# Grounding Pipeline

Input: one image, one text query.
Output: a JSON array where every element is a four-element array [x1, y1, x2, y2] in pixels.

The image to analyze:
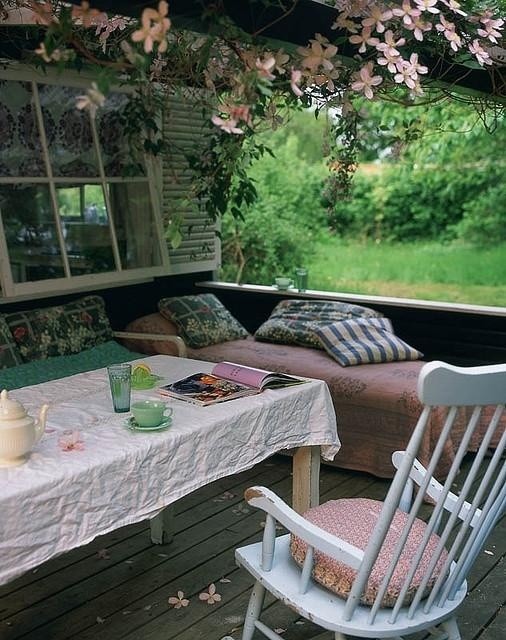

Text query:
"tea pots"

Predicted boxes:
[[1, 389, 50, 470]]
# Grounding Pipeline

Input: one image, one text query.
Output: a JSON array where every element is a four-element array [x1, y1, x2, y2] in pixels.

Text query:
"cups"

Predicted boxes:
[[130, 399, 173, 428], [107, 363, 132, 413]]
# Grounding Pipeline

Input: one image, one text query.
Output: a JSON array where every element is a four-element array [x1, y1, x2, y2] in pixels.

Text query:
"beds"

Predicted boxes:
[[121, 311, 506, 487]]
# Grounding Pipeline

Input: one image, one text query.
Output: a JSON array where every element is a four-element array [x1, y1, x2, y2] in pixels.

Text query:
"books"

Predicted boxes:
[[157, 361, 313, 408]]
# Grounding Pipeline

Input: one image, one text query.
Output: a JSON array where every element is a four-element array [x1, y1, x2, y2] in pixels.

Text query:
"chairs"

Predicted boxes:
[[0, 294, 186, 387], [220, 360, 506, 639]]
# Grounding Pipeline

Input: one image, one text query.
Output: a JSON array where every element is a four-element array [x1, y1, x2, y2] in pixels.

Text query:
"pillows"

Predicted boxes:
[[0, 295, 113, 368], [313, 316, 425, 368], [155, 292, 248, 349]]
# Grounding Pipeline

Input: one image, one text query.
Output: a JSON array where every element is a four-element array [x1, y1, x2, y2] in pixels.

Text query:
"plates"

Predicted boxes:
[[125, 416, 174, 432]]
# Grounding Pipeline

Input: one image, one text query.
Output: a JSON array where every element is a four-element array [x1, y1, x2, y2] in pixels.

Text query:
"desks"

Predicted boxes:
[[0, 355, 329, 546]]
